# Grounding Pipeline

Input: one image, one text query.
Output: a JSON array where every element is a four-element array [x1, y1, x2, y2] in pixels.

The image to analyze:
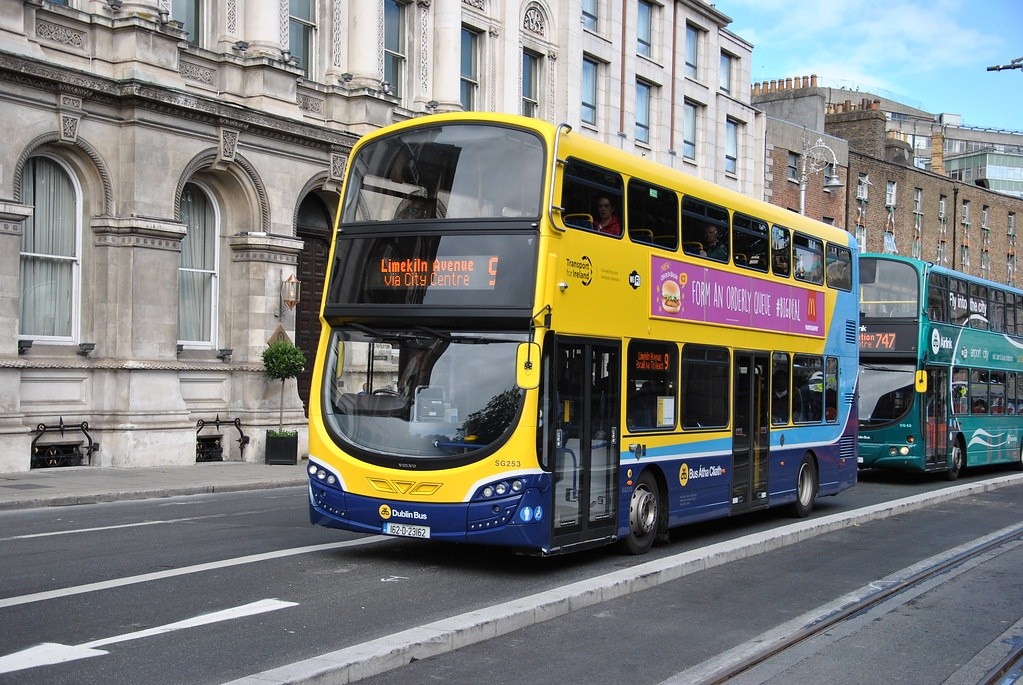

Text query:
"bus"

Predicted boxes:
[[305, 107, 862, 559], [808, 252, 1023, 481]]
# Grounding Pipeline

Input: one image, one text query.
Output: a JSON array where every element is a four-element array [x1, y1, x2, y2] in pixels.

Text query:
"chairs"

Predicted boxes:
[[566, 213, 768, 270]]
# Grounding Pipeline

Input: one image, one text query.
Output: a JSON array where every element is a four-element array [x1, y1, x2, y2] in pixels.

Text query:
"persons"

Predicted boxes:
[[401, 196, 434, 221], [592, 194, 621, 236], [602, 362, 675, 424], [754, 370, 802, 422], [699, 225, 729, 262]]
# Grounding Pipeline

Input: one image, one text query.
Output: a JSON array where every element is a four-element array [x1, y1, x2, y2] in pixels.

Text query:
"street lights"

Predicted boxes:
[[796, 136, 846, 218], [950, 177, 991, 271]]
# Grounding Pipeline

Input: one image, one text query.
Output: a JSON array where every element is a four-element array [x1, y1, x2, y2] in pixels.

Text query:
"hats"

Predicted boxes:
[[799, 370, 812, 383]]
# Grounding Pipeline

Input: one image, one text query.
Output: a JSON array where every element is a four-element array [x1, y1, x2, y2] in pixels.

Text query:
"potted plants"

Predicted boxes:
[[261, 341, 307, 465]]
